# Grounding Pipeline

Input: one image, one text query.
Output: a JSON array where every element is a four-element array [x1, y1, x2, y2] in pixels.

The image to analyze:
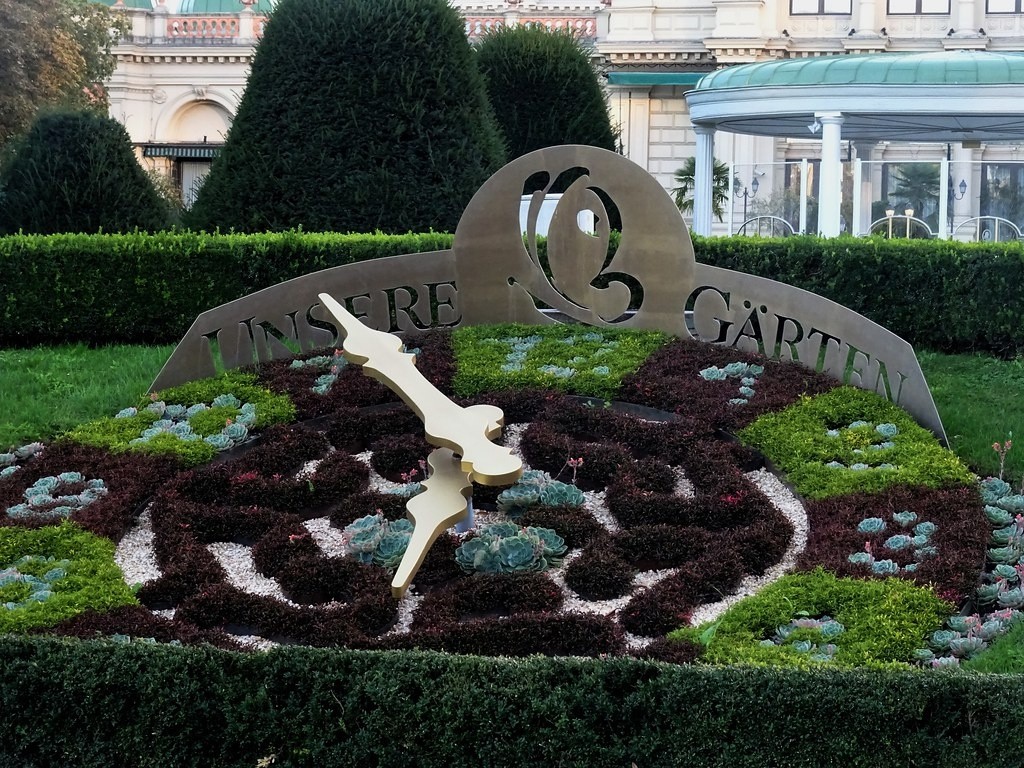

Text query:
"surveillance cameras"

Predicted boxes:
[[754, 169, 765, 177]]
